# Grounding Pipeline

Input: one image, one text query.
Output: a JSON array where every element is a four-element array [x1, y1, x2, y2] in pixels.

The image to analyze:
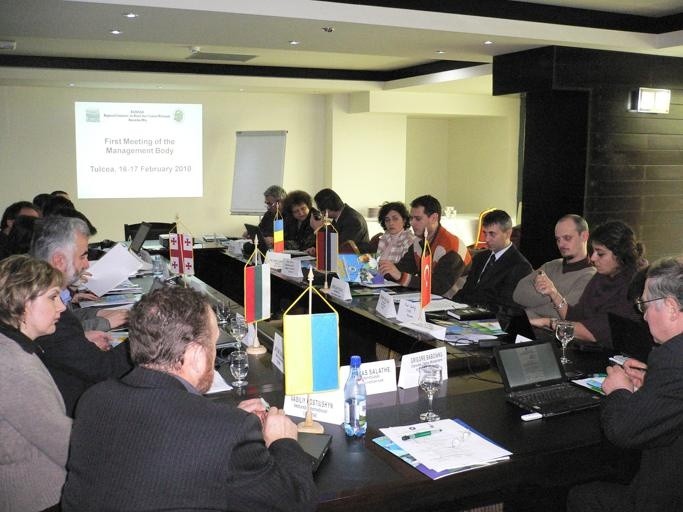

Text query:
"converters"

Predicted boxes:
[[478, 340, 502, 347]]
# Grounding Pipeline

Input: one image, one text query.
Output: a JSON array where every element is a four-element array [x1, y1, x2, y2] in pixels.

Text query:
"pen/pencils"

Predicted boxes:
[[586, 372, 607, 377], [628, 366, 648, 372], [403, 429, 442, 441], [259, 395, 271, 411], [535, 271, 544, 275]]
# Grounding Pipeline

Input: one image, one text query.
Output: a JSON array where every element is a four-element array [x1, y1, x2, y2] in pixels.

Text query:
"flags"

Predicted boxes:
[[169, 221, 195, 276], [244, 248, 271, 325], [420, 242, 431, 308], [283, 286, 340, 395], [313, 221, 339, 273]]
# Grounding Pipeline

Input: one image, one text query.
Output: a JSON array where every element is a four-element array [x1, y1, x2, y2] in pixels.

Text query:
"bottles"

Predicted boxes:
[[151, 251, 163, 277], [343, 356, 367, 438]]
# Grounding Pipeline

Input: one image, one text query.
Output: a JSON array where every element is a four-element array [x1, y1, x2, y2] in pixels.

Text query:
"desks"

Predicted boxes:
[[88, 236, 251, 285], [222, 242, 571, 355], [203, 348, 660, 512], [57, 254, 339, 401]]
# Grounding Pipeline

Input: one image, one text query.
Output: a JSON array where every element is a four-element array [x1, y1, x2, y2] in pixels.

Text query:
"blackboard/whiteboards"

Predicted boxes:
[[226, 128, 288, 216]]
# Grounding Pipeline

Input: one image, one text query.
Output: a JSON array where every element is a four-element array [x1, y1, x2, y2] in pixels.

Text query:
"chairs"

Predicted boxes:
[[124, 222, 177, 239]]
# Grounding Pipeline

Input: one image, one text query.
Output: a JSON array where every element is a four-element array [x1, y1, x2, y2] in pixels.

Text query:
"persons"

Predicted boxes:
[[565, 257, 683, 512], [452, 209, 535, 312], [61, 288, 319, 512], [530, 220, 656, 365], [513, 215, 598, 333], [1, 191, 133, 420], [244, 186, 369, 256], [379, 195, 472, 300], [0, 254, 73, 511], [372, 201, 418, 282]]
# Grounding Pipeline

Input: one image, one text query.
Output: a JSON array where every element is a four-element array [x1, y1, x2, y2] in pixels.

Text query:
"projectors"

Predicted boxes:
[[159, 234, 194, 248]]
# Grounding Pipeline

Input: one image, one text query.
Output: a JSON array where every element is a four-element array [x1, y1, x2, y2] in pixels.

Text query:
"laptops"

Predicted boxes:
[[244, 223, 268, 250], [129, 222, 153, 254], [492, 338, 606, 419], [486, 299, 556, 340]]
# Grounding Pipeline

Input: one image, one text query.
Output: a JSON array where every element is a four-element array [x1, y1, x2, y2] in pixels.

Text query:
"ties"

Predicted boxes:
[[480, 254, 495, 281]]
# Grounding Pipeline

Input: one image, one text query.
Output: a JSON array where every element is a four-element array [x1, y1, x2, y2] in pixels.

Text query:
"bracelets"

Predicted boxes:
[[554, 298, 567, 312], [550, 317, 560, 331]]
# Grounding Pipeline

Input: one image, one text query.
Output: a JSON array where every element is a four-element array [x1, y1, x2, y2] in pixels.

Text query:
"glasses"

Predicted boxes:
[[263, 201, 277, 209], [634, 296, 666, 312]]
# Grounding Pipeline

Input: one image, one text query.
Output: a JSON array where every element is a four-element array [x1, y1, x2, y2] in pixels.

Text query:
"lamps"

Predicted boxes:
[[628, 86, 671, 115]]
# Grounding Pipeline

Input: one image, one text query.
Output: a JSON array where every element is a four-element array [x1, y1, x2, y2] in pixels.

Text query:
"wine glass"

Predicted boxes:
[[230, 317, 249, 349], [217, 298, 232, 323], [419, 363, 443, 422], [229, 350, 249, 387], [555, 321, 576, 364]]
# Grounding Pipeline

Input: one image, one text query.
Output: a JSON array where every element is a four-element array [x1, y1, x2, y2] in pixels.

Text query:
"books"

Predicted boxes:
[[446, 306, 497, 320]]
